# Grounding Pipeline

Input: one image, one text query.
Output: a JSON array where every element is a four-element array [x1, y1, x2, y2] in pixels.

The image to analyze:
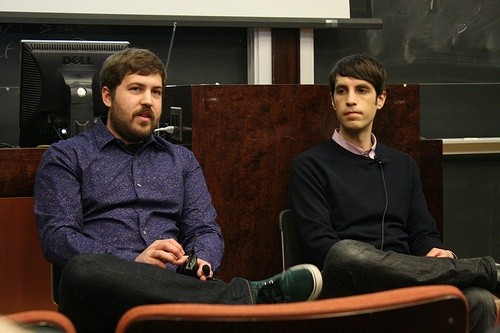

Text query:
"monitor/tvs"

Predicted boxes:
[[18, 40, 130, 147]]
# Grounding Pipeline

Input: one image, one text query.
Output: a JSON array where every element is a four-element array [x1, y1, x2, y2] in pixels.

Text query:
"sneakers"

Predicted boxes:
[[251, 264, 323, 305]]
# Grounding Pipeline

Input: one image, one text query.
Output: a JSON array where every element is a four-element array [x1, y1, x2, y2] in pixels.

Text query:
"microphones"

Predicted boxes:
[[203, 265, 210, 277], [378, 158, 389, 166], [165, 22, 177, 72]]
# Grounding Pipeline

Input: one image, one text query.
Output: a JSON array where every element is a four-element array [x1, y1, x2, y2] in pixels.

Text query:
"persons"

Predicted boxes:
[[289, 54, 500, 333], [34, 48, 324, 333]]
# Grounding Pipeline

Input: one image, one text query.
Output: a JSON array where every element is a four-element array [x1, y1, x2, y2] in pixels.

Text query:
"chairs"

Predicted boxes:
[[115, 285, 470, 333], [277, 209, 312, 274]]
